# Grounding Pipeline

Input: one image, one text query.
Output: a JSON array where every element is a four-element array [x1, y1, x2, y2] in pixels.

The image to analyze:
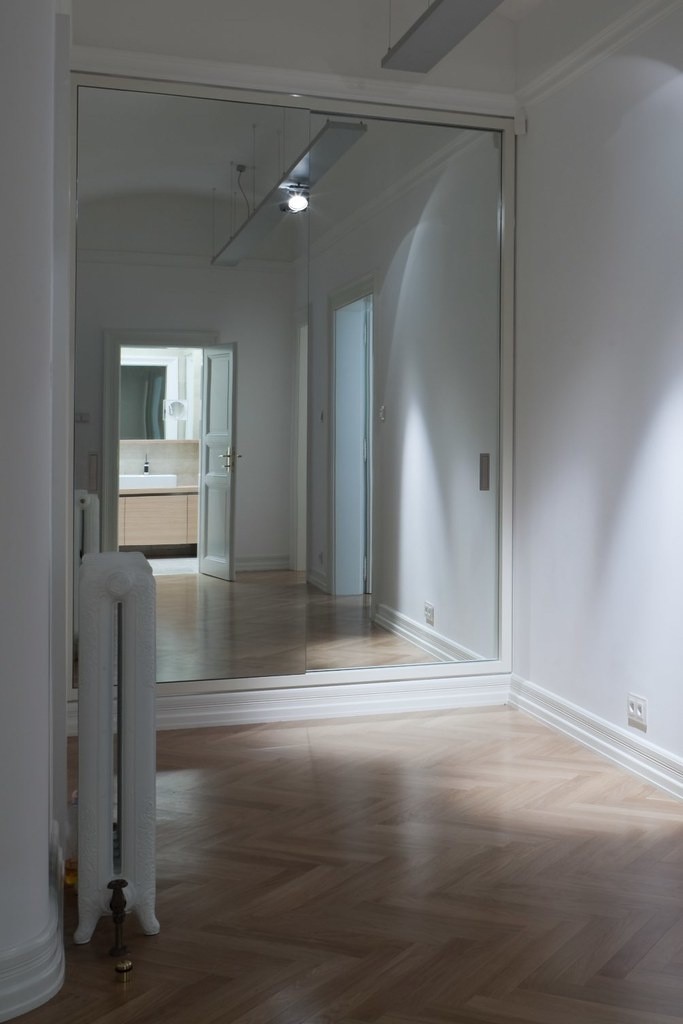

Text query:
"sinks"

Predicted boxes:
[[119, 475, 178, 490]]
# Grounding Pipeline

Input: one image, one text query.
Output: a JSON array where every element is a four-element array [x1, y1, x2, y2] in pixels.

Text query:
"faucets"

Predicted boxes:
[[143, 452, 150, 475]]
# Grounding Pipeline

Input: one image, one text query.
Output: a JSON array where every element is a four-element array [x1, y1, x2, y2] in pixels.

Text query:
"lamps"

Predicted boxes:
[[210, 106, 368, 268]]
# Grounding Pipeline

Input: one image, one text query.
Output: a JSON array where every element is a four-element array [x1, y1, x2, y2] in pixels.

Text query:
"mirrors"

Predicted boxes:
[[71, 69, 502, 687], [120, 366, 167, 440]]
